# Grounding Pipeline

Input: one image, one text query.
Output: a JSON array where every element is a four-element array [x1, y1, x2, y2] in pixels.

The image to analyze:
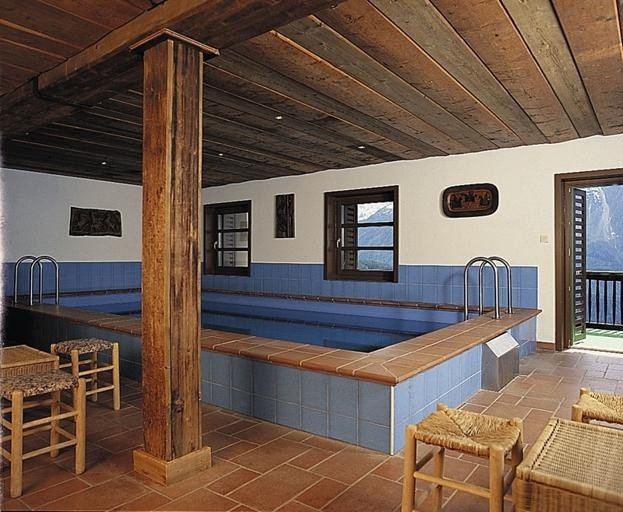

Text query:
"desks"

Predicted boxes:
[[1, 343, 60, 380], [512, 416, 623, 511]]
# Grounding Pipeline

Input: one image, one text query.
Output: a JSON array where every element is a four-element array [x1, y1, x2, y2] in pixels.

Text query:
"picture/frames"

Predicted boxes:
[[442, 182, 498, 217]]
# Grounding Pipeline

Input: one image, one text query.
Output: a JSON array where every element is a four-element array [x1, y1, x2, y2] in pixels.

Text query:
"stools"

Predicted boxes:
[[571, 385, 623, 424], [1, 369, 86, 499], [50, 338, 123, 411], [401, 404, 522, 512]]
